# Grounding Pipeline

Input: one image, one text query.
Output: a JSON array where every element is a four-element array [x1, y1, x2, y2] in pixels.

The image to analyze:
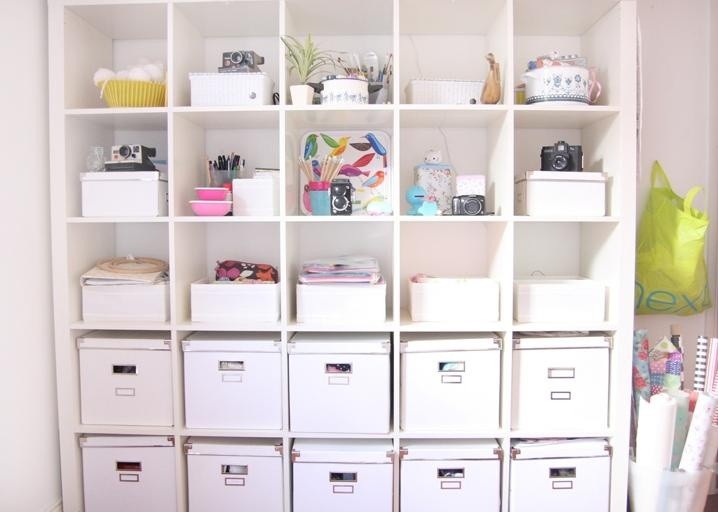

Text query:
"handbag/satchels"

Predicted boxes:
[[634, 156, 713, 316]]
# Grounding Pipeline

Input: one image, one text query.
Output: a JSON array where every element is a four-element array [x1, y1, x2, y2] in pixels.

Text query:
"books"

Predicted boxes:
[[79, 260, 162, 284]]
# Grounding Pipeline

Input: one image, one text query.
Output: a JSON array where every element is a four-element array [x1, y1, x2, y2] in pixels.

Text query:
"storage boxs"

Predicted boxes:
[[79, 171, 168, 217], [232, 178, 280, 216], [514, 170, 607, 216]]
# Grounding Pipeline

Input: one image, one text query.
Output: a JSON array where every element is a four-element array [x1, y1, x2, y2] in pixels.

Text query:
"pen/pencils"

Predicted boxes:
[[338, 54, 393, 80], [209, 151, 246, 171], [300, 156, 343, 182]]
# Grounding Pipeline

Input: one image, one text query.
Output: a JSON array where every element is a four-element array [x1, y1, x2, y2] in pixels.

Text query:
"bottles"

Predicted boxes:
[[224, 184, 232, 200]]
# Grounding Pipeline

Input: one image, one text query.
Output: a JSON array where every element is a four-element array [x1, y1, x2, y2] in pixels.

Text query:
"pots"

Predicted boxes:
[[520, 65, 594, 107], [303, 77, 384, 106]]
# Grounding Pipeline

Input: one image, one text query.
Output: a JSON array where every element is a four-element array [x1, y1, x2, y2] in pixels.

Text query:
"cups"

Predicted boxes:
[[309, 181, 329, 191], [311, 191, 332, 216]]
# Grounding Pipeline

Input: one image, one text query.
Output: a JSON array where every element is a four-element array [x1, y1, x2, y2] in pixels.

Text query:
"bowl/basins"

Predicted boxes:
[[195, 187, 227, 200], [188, 200, 233, 216]]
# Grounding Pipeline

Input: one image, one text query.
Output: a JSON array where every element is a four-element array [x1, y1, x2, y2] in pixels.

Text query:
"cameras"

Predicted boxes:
[[222, 51, 266, 66], [539, 140, 584, 172], [329, 178, 353, 215], [111, 144, 156, 164], [451, 194, 485, 216]]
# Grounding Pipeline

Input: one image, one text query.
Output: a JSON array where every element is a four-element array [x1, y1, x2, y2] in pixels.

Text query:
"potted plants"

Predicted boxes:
[[280, 33, 349, 105]]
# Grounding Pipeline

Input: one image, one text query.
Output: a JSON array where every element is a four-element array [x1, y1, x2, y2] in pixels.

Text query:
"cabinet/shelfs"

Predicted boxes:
[[48, 0, 638, 512]]
